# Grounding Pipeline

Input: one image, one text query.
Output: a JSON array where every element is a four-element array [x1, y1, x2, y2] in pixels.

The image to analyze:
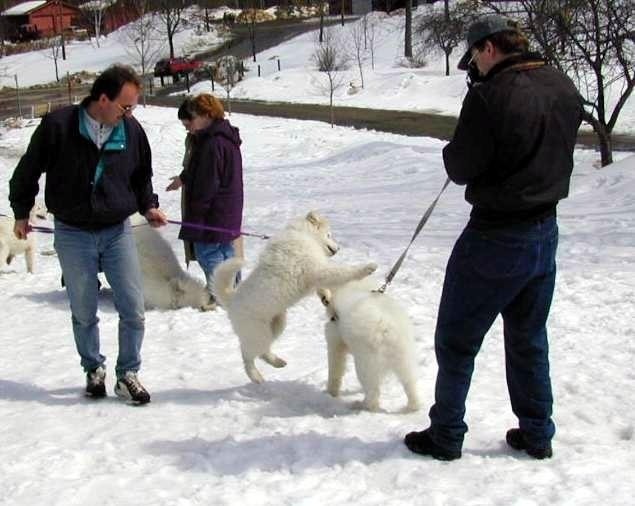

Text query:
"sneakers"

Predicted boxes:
[[405, 430, 461, 460], [506, 428, 553, 460], [115, 370, 151, 404], [84, 366, 108, 399]]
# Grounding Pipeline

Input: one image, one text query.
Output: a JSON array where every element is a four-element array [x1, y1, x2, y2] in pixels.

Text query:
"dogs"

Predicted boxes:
[[128, 211, 207, 312], [208, 210, 377, 385], [0, 200, 49, 275], [321, 269, 423, 413]]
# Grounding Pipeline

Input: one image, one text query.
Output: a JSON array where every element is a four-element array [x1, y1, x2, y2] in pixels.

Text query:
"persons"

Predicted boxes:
[[9, 61, 167, 407], [166, 93, 245, 314], [403, 15, 588, 465], [177, 94, 245, 287]]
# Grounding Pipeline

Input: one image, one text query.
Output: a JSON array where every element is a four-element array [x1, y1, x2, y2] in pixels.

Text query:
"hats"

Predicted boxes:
[[456, 14, 522, 72]]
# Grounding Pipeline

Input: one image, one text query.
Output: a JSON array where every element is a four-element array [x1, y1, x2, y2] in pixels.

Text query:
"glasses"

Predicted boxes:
[[110, 98, 137, 113], [466, 46, 481, 71]]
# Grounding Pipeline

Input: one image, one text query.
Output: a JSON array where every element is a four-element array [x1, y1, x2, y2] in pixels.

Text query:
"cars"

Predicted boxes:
[[154, 56, 203, 80]]
